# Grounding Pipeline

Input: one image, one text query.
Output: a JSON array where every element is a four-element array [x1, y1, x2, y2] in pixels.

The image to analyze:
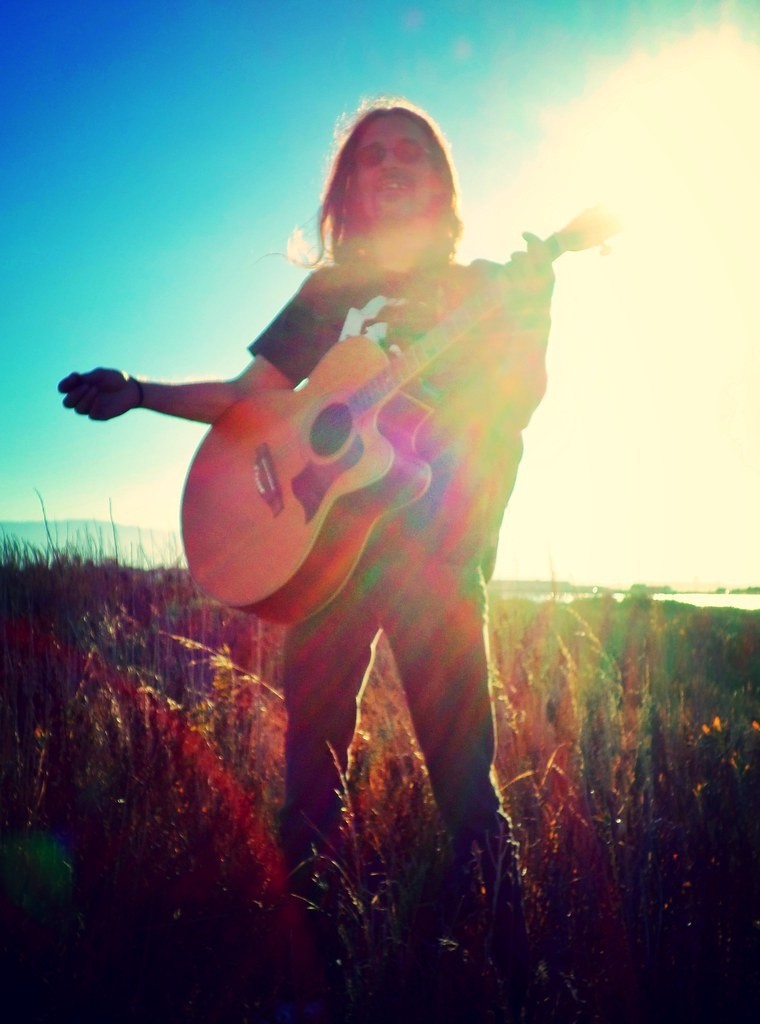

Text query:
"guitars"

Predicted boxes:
[[178, 200, 628, 623]]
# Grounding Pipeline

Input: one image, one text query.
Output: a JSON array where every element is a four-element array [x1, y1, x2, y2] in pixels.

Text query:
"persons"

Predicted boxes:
[[58, 102, 555, 1024]]
